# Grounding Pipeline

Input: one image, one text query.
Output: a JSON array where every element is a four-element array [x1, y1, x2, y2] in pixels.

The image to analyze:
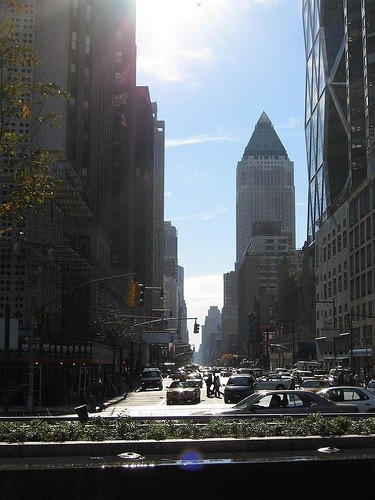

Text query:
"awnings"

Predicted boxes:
[[348, 348, 373, 357]]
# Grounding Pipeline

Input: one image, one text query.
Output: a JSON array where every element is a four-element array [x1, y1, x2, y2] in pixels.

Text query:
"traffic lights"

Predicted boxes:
[[127, 281, 145, 307], [194, 323, 199, 333]]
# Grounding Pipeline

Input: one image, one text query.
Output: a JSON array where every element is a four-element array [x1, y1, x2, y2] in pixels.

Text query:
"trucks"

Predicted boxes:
[[296, 360, 323, 371]]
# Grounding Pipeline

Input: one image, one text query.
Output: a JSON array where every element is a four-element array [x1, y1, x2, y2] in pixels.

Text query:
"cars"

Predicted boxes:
[[165, 368, 204, 404], [139, 368, 164, 390], [190, 389, 359, 420], [214, 366, 375, 414]]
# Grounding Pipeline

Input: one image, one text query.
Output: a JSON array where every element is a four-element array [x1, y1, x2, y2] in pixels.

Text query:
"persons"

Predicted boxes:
[[368, 377, 375, 388], [90, 375, 106, 413], [288, 378, 295, 401], [262, 395, 281, 408], [338, 372, 360, 386], [204, 372, 220, 398]]
